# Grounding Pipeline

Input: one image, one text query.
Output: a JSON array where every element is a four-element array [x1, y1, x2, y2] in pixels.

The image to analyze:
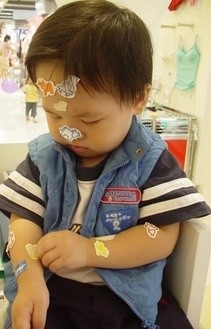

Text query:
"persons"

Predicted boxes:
[[21, 74, 40, 122], [12, 50, 27, 88], [0, 35, 13, 80], [0, 0, 210, 329]]
